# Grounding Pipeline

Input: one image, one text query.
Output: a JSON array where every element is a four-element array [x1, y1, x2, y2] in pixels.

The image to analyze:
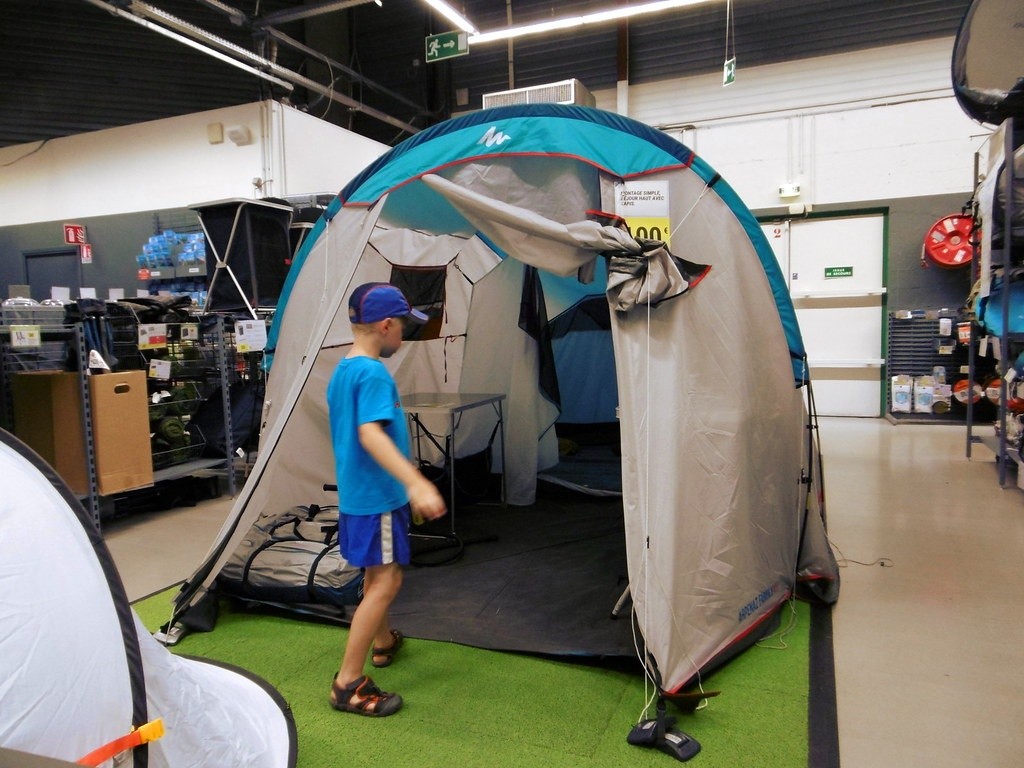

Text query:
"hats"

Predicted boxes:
[[349, 281, 427, 324]]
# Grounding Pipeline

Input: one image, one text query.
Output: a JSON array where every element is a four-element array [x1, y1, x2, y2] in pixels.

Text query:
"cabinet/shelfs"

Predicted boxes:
[[0, 294, 277, 529], [136, 212, 208, 294], [965, 119, 1024, 490], [888, 313, 997, 425]]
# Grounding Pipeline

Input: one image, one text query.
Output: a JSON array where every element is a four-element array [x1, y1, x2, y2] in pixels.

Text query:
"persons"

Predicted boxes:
[[327, 283, 450, 719]]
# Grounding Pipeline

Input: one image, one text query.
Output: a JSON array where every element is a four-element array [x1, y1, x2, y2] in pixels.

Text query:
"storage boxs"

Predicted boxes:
[[187, 198, 293, 312], [289, 204, 328, 258]]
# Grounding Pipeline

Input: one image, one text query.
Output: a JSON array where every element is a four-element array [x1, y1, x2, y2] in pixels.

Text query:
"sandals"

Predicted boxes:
[[329, 668, 403, 718], [371, 625, 404, 668]]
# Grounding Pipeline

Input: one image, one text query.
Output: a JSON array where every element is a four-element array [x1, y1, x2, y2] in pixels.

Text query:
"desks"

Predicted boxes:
[[398, 393, 509, 543]]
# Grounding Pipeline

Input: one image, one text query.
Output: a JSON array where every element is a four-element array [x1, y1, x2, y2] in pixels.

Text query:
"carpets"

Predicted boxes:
[[128, 580, 837, 768]]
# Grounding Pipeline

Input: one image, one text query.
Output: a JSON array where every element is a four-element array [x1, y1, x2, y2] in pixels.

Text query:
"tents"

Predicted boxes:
[[1, 427, 298, 768], [153, 104, 841, 763]]
